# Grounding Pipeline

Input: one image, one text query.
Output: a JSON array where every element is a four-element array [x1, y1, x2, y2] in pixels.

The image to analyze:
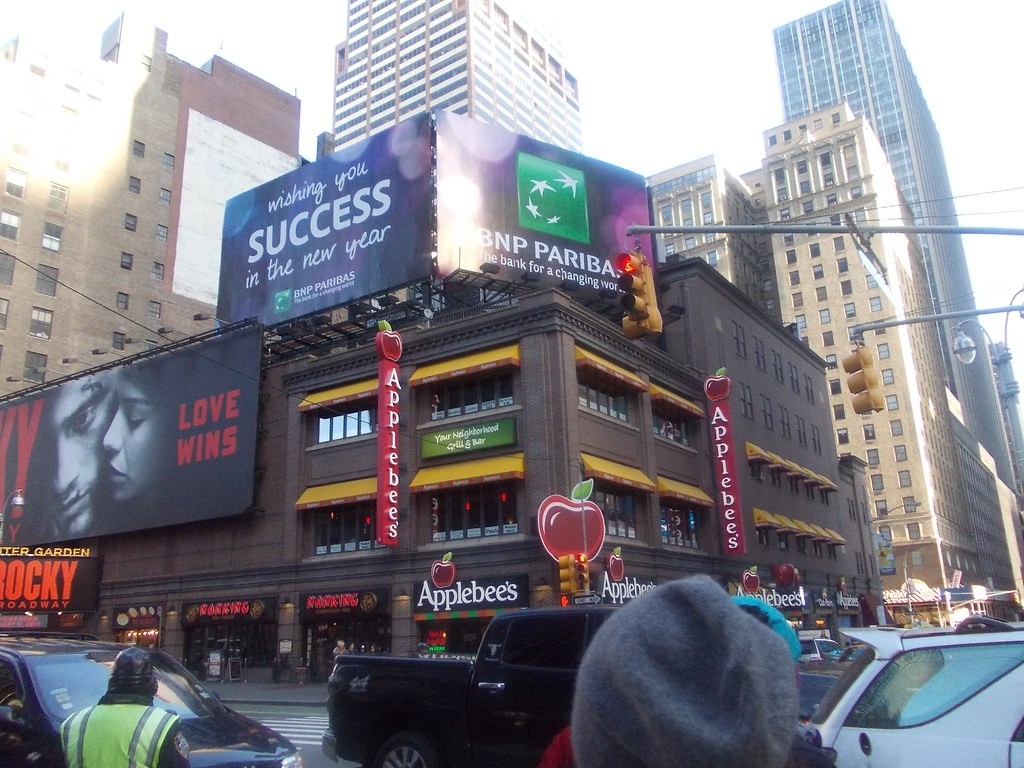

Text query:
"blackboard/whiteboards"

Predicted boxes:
[[226, 657, 242, 681]]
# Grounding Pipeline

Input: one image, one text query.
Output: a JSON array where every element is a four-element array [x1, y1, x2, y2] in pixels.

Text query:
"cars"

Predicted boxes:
[[796, 614, 1024, 767]]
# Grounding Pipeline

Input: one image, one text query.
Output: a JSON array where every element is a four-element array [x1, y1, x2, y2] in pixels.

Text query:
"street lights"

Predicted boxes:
[[949, 285, 1024, 513], [860, 484, 922, 627], [904, 535, 933, 623]]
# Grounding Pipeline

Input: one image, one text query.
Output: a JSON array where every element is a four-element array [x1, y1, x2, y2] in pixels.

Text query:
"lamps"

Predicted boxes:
[[157, 326, 192, 337], [5, 376, 40, 384], [91, 349, 126, 358], [62, 357, 96, 367], [558, 279, 579, 294], [125, 337, 161, 347], [466, 262, 500, 286], [276, 324, 297, 338], [311, 314, 332, 329], [349, 301, 371, 317], [377, 295, 408, 310], [584, 288, 618, 308], [33, 367, 68, 376], [192, 312, 236, 327], [662, 305, 685, 326], [500, 270, 541, 300]]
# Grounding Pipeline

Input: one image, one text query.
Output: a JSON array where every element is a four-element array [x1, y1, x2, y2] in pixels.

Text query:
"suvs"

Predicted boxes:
[[0, 626, 306, 768]]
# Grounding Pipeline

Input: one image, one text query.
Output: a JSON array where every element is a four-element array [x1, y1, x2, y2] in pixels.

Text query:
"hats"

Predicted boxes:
[[571, 572, 801, 768], [730, 593, 800, 663]]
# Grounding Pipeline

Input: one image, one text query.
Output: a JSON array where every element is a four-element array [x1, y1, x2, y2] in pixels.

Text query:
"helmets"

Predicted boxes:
[[108, 648, 157, 695]]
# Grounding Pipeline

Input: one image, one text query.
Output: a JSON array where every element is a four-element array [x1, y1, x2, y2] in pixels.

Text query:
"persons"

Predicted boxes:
[[820, 629, 828, 639], [370, 643, 376, 652], [539, 573, 839, 768], [332, 639, 349, 656], [42, 356, 225, 539], [411, 643, 428, 658], [59, 646, 191, 768], [347, 641, 359, 653]]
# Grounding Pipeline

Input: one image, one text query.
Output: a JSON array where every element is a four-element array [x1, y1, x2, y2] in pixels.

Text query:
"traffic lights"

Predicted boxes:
[[1018, 512, 1024, 542], [574, 554, 590, 589], [558, 554, 577, 595], [620, 264, 664, 341], [614, 250, 650, 320], [842, 346, 886, 415], [559, 594, 570, 607]]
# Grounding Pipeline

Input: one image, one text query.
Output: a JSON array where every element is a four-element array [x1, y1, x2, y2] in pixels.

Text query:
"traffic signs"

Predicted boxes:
[[573, 594, 602, 606]]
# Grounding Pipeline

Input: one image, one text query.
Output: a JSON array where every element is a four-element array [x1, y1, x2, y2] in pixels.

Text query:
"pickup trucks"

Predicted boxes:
[[322, 604, 854, 768]]
